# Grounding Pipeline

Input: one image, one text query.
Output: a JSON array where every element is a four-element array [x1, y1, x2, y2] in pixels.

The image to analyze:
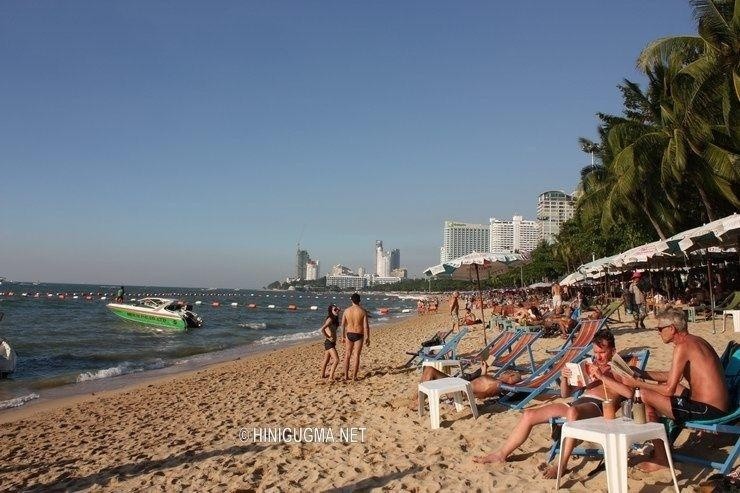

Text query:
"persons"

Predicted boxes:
[[448, 290, 462, 334], [414, 293, 440, 313], [320, 303, 341, 382], [621, 306, 730, 473], [341, 292, 371, 382], [116, 285, 125, 303], [471, 328, 635, 482], [407, 359, 523, 407], [459, 267, 740, 339]]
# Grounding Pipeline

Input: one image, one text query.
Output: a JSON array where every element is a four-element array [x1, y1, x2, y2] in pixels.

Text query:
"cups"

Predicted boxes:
[[602, 400, 615, 419]]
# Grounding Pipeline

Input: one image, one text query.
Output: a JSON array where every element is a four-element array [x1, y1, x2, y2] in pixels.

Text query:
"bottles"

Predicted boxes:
[[631, 386, 646, 424]]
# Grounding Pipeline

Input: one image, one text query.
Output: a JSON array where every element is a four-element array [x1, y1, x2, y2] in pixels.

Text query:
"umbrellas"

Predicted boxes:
[[558, 211, 740, 334], [422, 244, 533, 345]]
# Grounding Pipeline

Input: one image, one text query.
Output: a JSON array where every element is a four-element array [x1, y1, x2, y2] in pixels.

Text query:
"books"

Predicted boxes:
[[563, 356, 601, 390], [607, 351, 641, 380]]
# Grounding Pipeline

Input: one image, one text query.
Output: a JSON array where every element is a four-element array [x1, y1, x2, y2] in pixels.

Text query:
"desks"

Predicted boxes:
[[418, 377, 479, 428], [556, 416, 682, 493], [653, 304, 706, 323], [722, 310, 740, 333]]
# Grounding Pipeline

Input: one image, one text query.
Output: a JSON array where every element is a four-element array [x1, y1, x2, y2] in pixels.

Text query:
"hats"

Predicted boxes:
[[631, 272, 642, 279]]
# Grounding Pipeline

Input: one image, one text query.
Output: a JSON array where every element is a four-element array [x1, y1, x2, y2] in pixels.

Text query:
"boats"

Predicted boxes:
[[106, 298, 202, 333]]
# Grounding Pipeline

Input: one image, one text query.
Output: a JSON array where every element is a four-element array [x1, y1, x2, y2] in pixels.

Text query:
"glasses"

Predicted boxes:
[[334, 308, 339, 311], [657, 325, 671, 332]]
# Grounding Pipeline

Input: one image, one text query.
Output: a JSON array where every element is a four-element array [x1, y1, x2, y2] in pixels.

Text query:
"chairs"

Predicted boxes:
[[404, 317, 609, 410], [706, 292, 740, 321], [553, 301, 623, 336], [547, 350, 650, 476], [548, 304, 607, 335], [628, 340, 740, 476]]
